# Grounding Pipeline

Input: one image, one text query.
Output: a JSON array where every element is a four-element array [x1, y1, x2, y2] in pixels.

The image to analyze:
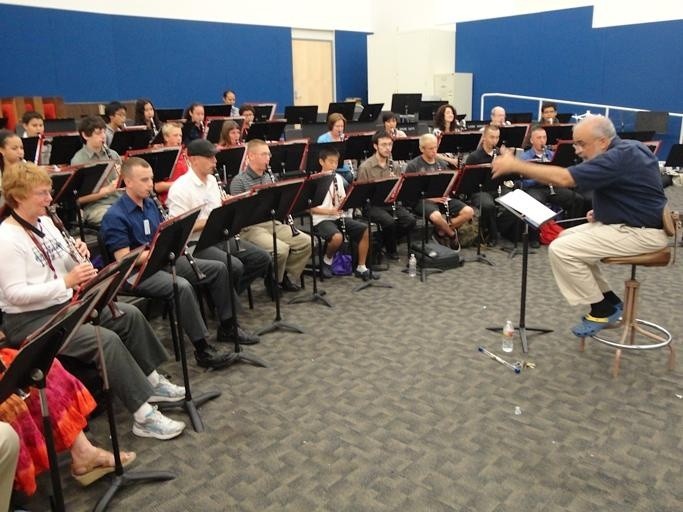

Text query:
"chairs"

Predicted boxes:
[[578, 203, 679, 378]]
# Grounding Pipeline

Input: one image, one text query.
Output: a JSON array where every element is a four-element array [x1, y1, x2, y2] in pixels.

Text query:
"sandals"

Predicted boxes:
[[571, 301, 623, 339]]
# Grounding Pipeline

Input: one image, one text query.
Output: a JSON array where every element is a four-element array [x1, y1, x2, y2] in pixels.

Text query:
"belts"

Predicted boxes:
[[627, 223, 656, 228]]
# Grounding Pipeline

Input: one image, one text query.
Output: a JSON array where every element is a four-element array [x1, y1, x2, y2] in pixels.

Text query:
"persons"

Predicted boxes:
[[466, 126, 516, 247], [519, 126, 584, 249], [430, 106, 466, 169], [19, 112, 48, 146], [491, 114, 669, 338], [97, 157, 261, 371], [102, 102, 128, 147], [524, 102, 565, 154], [0, 421, 20, 512], [0, 159, 189, 440], [230, 140, 313, 299], [165, 139, 274, 321], [0, 351, 135, 490], [182, 104, 206, 147], [382, 113, 408, 176], [0, 132, 25, 211], [222, 90, 241, 119], [69, 116, 127, 226], [136, 100, 163, 147], [478, 106, 512, 137], [215, 120, 249, 184], [317, 113, 354, 191], [237, 103, 256, 144], [357, 131, 413, 272], [406, 134, 474, 254], [153, 122, 189, 208], [305, 147, 371, 282]]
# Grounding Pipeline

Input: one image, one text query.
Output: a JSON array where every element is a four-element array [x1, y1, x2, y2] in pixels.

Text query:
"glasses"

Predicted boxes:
[[571, 137, 603, 149], [117, 113, 126, 116], [253, 152, 272, 158]]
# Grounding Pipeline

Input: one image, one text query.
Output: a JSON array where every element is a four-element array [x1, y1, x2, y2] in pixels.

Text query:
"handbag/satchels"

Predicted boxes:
[[538, 219, 565, 245]]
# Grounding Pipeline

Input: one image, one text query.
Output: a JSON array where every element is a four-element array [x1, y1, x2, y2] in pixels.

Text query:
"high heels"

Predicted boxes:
[[70, 448, 136, 487]]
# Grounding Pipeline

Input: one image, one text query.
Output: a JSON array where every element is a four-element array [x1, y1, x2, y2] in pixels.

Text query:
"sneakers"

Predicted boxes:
[[530, 241, 539, 248], [134, 380, 188, 440]]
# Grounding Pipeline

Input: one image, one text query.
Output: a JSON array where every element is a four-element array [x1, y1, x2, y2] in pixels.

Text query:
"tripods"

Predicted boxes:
[[92, 319, 176, 512], [147, 259, 221, 433], [351, 203, 394, 293], [401, 195, 443, 283], [288, 203, 336, 307], [486, 223, 554, 354], [252, 214, 306, 336], [465, 187, 496, 266], [203, 234, 271, 373], [500, 176, 537, 259]]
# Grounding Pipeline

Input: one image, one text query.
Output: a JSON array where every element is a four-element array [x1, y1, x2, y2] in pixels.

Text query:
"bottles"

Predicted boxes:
[[409, 254, 417, 277], [503, 319, 514, 352]]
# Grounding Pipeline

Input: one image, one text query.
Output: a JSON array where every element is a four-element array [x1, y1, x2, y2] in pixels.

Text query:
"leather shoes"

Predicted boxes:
[[194, 324, 261, 366], [267, 232, 461, 300]]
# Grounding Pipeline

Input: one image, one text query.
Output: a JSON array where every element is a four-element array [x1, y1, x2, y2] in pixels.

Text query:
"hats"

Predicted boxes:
[[189, 139, 218, 157]]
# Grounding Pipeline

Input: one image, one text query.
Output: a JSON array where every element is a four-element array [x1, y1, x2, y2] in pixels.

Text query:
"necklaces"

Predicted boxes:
[[19, 223, 57, 280]]
